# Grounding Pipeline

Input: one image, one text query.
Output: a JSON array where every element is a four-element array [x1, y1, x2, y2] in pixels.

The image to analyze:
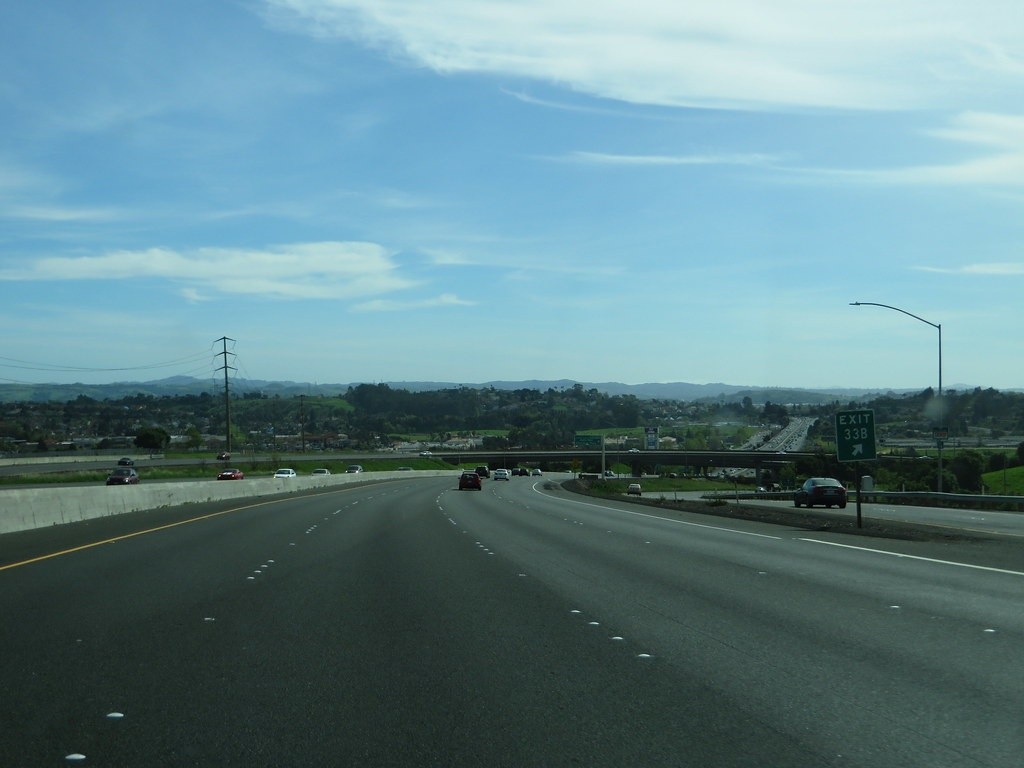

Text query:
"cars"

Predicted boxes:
[[272, 469, 296, 478], [532, 469, 542, 476], [312, 469, 330, 475], [119, 457, 134, 465], [756, 487, 766, 492], [458, 473, 482, 490], [105, 468, 139, 486], [513, 467, 531, 476], [217, 452, 231, 460], [398, 467, 414, 471], [494, 469, 511, 480], [217, 469, 243, 480], [345, 464, 362, 473], [629, 447, 640, 453], [794, 478, 846, 508], [420, 450, 432, 455]]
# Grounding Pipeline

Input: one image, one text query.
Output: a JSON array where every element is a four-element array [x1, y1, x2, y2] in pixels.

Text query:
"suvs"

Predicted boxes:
[[475, 466, 490, 478], [766, 483, 781, 492]]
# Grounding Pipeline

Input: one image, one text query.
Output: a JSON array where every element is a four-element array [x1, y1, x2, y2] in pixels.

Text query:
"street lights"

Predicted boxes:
[[851, 301, 942, 502]]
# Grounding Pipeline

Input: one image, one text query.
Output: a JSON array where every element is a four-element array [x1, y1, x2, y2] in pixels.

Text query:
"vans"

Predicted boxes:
[[627, 483, 641, 494]]
